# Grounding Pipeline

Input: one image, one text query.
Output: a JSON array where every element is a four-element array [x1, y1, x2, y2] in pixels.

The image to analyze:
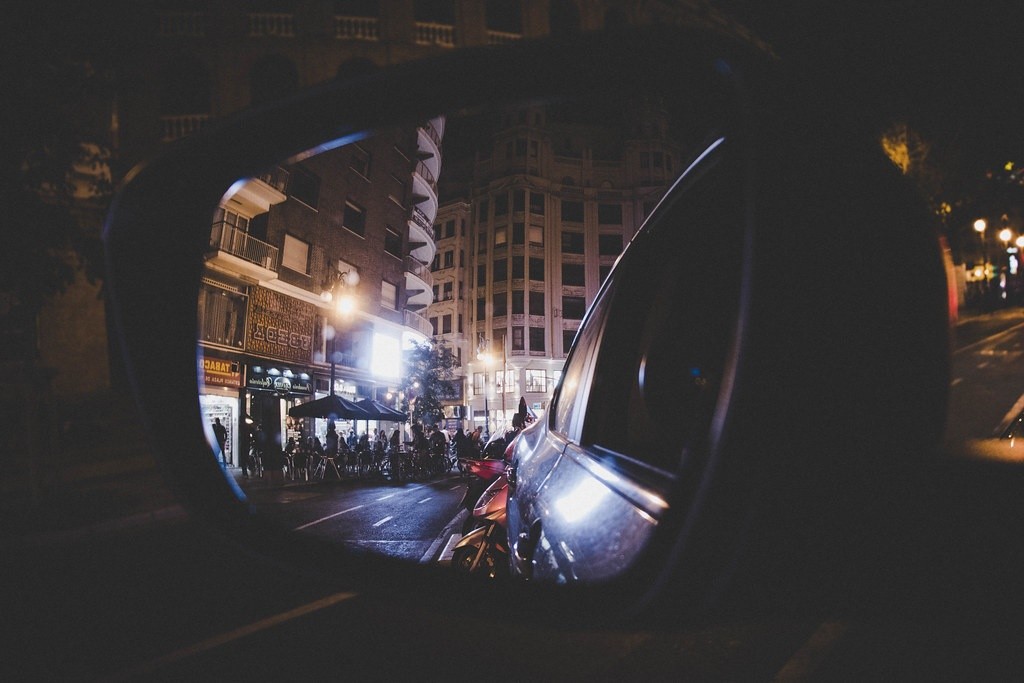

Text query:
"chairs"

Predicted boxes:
[[280, 450, 389, 482]]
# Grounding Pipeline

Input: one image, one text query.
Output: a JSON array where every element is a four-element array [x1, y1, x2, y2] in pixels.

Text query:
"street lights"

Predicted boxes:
[[319, 257, 350, 455], [475, 334, 493, 438]]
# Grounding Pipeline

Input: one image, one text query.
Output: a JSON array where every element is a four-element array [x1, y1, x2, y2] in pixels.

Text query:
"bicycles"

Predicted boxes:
[[346, 446, 465, 480]]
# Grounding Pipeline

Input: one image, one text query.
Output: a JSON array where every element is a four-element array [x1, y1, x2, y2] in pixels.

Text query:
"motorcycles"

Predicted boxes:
[[448, 468, 512, 582]]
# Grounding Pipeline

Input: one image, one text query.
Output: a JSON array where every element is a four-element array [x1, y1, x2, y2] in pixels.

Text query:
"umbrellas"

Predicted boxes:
[[352, 397, 409, 435], [288, 394, 371, 421]]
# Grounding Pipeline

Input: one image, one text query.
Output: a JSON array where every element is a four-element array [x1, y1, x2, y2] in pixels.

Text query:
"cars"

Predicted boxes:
[[463, 428, 516, 508]]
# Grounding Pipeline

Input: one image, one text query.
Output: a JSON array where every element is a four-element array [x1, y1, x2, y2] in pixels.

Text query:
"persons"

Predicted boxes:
[[238, 412, 485, 488], [211, 417, 227, 470]]
